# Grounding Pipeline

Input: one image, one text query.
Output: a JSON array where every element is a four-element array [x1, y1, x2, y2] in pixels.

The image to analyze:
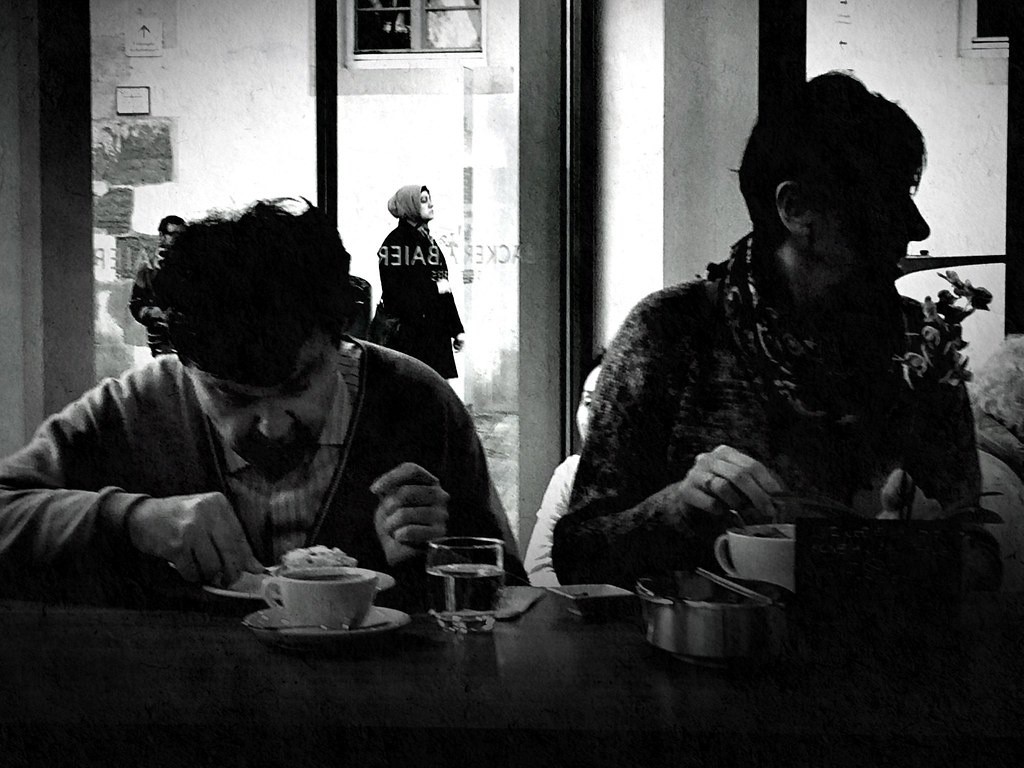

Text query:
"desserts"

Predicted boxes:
[[281, 546, 358, 572]]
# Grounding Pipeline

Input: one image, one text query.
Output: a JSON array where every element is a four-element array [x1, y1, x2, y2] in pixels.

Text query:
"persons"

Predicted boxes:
[[0, 195, 530, 621], [550, 71, 988, 588], [128, 215, 185, 359], [367, 184, 464, 380], [523, 344, 606, 589]]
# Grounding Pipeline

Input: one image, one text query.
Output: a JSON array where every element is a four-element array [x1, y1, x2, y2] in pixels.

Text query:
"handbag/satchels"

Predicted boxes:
[[368, 302, 405, 346]]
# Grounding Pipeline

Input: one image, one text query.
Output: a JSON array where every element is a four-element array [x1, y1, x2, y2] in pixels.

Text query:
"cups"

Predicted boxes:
[[425, 537, 507, 632], [714, 524, 797, 594], [261, 566, 380, 630]]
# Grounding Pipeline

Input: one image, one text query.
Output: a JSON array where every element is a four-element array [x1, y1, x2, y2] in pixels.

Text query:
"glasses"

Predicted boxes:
[[166, 230, 178, 236], [421, 195, 428, 202]]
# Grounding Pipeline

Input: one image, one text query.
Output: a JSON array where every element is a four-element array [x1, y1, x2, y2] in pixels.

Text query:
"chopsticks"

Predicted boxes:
[[694, 565, 774, 607]]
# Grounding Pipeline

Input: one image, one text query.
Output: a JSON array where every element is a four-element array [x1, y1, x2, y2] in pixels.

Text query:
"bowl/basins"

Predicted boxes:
[[635, 571, 792, 664]]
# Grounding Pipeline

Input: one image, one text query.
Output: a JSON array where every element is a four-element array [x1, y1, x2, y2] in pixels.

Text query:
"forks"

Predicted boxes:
[[242, 618, 392, 631]]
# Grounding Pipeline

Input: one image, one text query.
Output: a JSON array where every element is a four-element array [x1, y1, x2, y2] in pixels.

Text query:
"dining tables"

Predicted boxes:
[[0, 583, 1023, 738]]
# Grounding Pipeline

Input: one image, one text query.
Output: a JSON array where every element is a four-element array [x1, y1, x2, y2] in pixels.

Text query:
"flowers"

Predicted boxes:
[[770, 267, 1007, 592]]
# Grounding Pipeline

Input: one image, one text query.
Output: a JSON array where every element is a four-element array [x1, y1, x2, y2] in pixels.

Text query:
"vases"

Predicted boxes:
[[794, 514, 964, 668]]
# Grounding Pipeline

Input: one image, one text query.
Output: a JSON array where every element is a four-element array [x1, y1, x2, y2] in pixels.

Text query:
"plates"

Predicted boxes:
[[542, 583, 634, 622], [199, 565, 398, 607], [243, 605, 411, 649]]
